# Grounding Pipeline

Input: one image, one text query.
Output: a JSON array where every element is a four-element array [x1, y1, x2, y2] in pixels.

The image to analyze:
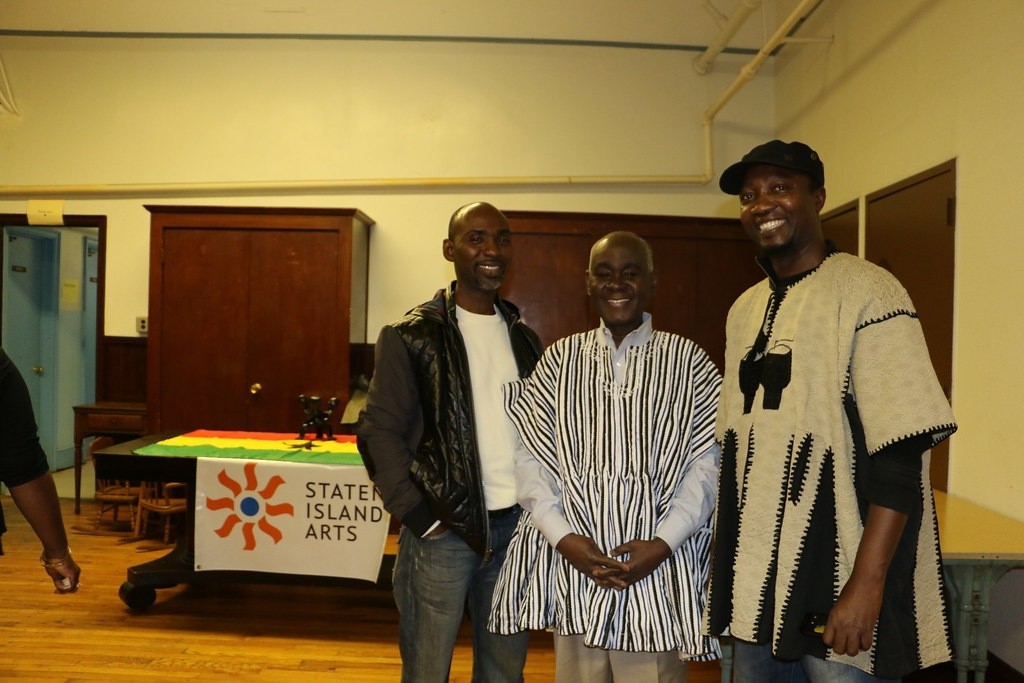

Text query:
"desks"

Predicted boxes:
[[927, 483, 1023, 682], [92, 426, 409, 609]]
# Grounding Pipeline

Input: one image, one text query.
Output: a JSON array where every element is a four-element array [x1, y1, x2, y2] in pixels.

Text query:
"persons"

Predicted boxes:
[[0, 346, 80, 594], [701, 140, 960, 683], [486, 232, 724, 683], [356, 201, 545, 683]]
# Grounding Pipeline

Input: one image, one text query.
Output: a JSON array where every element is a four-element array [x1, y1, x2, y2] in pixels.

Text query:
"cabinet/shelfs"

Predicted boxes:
[[74, 400, 146, 518], [488, 206, 776, 386], [143, 202, 377, 497]]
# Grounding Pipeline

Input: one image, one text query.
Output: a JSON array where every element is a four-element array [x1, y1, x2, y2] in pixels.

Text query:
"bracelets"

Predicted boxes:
[[39, 547, 72, 568]]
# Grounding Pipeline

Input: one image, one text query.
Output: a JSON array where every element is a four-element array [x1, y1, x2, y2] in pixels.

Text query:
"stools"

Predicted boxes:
[[89, 438, 140, 529], [133, 468, 188, 544]]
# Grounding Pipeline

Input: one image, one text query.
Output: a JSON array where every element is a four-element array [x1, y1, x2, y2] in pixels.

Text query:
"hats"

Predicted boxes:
[[719, 140, 825, 195]]
